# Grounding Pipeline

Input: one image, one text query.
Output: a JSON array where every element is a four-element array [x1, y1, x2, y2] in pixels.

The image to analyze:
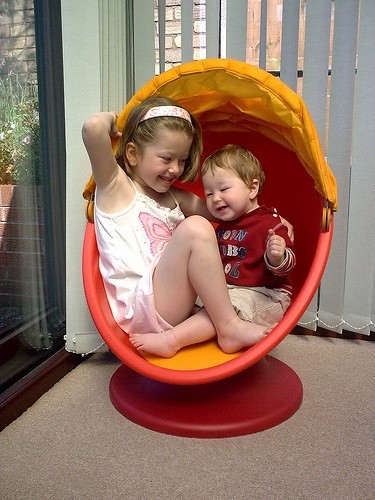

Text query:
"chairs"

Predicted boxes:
[[81, 58, 338, 438]]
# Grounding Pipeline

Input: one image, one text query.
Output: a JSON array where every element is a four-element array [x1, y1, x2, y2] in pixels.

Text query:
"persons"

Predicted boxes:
[[82, 94, 280, 354], [127, 144, 297, 360]]
